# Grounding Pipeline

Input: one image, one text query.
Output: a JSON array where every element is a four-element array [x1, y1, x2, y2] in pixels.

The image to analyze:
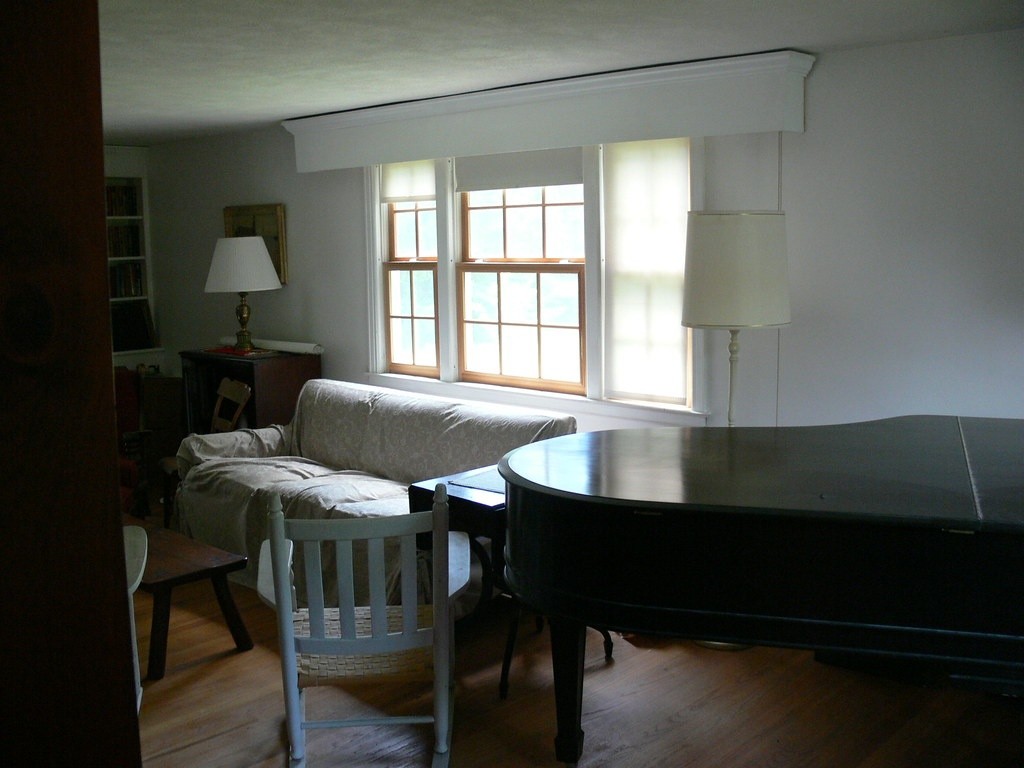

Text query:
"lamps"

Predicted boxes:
[[204, 236, 283, 350], [682, 210, 788, 426]]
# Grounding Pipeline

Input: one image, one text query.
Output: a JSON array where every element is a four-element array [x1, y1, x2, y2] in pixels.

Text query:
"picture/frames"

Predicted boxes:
[[222, 203, 288, 285]]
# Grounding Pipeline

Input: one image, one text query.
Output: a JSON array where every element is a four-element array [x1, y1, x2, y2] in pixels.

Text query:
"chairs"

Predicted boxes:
[[158, 377, 250, 527], [266, 484, 457, 767]]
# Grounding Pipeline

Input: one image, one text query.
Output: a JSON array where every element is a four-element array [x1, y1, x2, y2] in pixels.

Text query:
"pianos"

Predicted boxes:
[[497, 410, 1024, 763]]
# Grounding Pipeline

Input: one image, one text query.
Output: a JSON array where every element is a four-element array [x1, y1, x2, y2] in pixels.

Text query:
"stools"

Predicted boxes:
[[120, 509, 254, 680]]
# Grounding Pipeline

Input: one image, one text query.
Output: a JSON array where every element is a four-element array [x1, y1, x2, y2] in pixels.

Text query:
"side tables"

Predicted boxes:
[[410, 462, 614, 694]]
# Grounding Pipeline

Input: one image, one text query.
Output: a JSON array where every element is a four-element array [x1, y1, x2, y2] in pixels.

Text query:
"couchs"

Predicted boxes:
[[172, 378, 576, 626]]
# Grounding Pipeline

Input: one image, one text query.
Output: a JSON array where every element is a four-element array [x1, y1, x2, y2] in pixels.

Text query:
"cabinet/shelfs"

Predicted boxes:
[[179, 345, 321, 434], [104, 174, 163, 355]]
